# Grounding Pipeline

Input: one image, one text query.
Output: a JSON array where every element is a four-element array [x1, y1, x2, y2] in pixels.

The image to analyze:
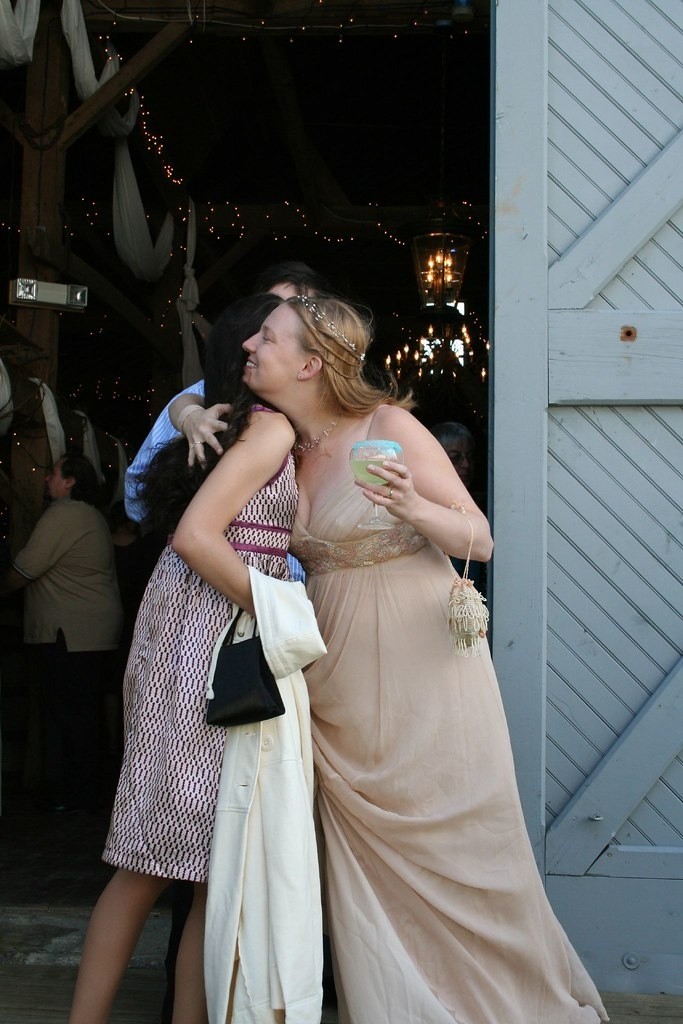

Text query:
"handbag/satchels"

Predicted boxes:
[[206, 607, 285, 728], [443, 501, 489, 658]]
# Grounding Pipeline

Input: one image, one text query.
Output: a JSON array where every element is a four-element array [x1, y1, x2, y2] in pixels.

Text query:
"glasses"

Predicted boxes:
[[451, 454, 474, 465]]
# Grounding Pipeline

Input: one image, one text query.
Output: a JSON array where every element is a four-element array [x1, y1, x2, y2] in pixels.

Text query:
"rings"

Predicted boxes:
[[388, 488, 393, 499], [191, 441, 201, 448]]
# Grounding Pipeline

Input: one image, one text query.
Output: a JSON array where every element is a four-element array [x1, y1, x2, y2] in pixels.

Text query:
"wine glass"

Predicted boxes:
[[348, 440, 404, 531]]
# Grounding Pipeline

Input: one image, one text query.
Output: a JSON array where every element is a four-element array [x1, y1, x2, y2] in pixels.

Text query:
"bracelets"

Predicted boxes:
[[176, 405, 204, 432]]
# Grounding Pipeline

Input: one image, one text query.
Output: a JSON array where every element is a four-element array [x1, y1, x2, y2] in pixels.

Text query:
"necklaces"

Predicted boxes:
[[295, 418, 337, 452]]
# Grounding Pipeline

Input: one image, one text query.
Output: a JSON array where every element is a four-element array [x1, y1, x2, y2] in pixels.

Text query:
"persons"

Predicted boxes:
[[167, 297, 610, 1024], [69, 293, 298, 1024], [429, 422, 487, 593], [0, 453, 128, 816], [123, 262, 322, 523]]
